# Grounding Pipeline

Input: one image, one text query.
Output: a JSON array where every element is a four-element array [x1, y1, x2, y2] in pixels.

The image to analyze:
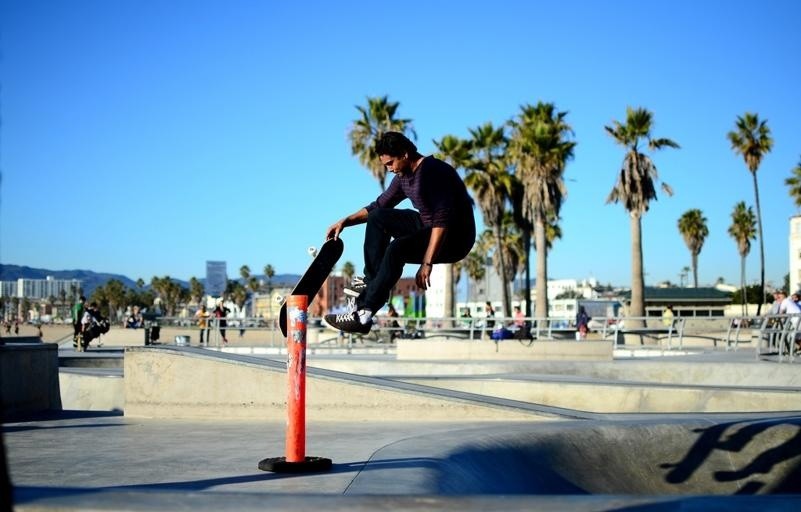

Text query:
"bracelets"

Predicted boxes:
[[422, 262, 432, 266]]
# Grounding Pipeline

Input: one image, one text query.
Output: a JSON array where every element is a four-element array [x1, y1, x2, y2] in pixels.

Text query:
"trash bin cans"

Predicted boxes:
[[151, 326, 162, 343]]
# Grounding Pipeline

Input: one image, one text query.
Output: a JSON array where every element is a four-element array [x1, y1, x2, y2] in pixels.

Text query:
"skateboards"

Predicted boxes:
[[279, 238, 344, 339]]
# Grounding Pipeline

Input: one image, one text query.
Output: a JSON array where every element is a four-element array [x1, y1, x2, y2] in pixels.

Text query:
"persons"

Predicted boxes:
[[733, 289, 801, 356], [507, 307, 525, 332], [574, 306, 592, 341], [463, 305, 474, 322], [486, 301, 496, 338], [663, 305, 675, 327], [1, 295, 255, 352], [386, 303, 405, 343], [318, 131, 478, 335]]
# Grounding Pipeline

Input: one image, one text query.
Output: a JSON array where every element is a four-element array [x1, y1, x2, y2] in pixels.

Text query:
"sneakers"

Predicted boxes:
[[321, 311, 375, 337], [342, 276, 367, 297]]
